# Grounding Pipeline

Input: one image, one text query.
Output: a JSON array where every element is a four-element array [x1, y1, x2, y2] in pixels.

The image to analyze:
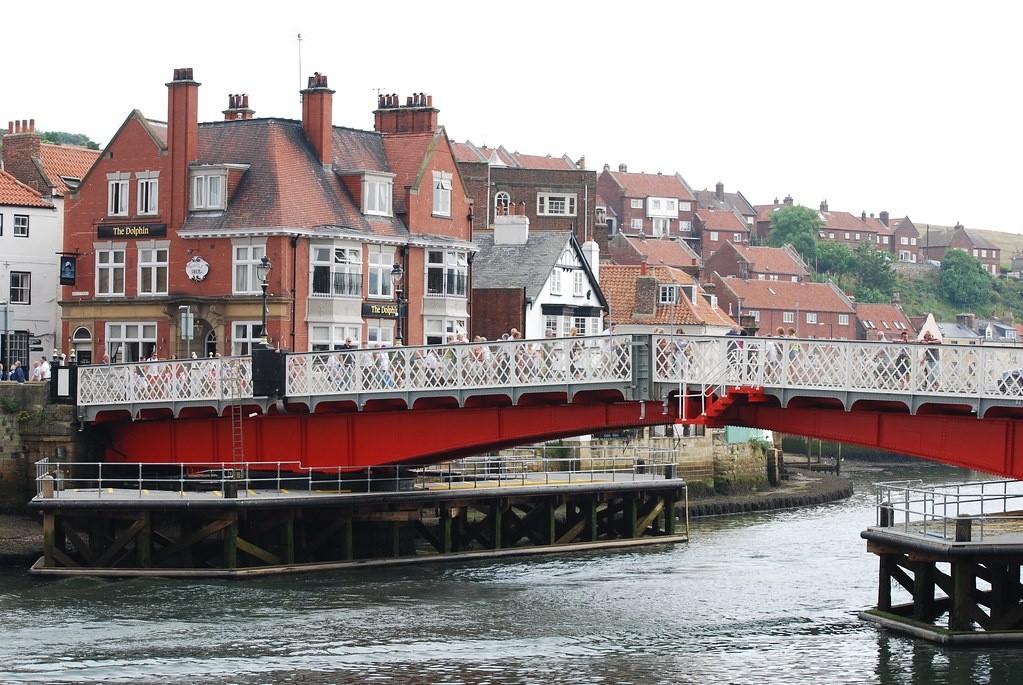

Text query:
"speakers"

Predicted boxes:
[[251, 349, 275, 381]]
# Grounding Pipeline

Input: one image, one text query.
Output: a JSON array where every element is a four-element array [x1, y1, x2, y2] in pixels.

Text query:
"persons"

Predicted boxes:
[[724, 324, 800, 383], [871, 330, 942, 390], [0, 322, 694, 403]]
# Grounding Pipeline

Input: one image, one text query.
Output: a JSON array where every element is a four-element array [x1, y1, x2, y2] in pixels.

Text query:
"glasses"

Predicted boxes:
[[348, 341, 351, 342]]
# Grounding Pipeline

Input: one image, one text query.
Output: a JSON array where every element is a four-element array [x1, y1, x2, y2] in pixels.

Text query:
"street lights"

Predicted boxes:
[[256, 255, 273, 344], [390, 261, 404, 338]]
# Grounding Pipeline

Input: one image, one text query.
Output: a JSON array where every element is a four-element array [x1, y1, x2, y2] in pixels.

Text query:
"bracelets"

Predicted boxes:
[[876, 353, 879, 356]]
